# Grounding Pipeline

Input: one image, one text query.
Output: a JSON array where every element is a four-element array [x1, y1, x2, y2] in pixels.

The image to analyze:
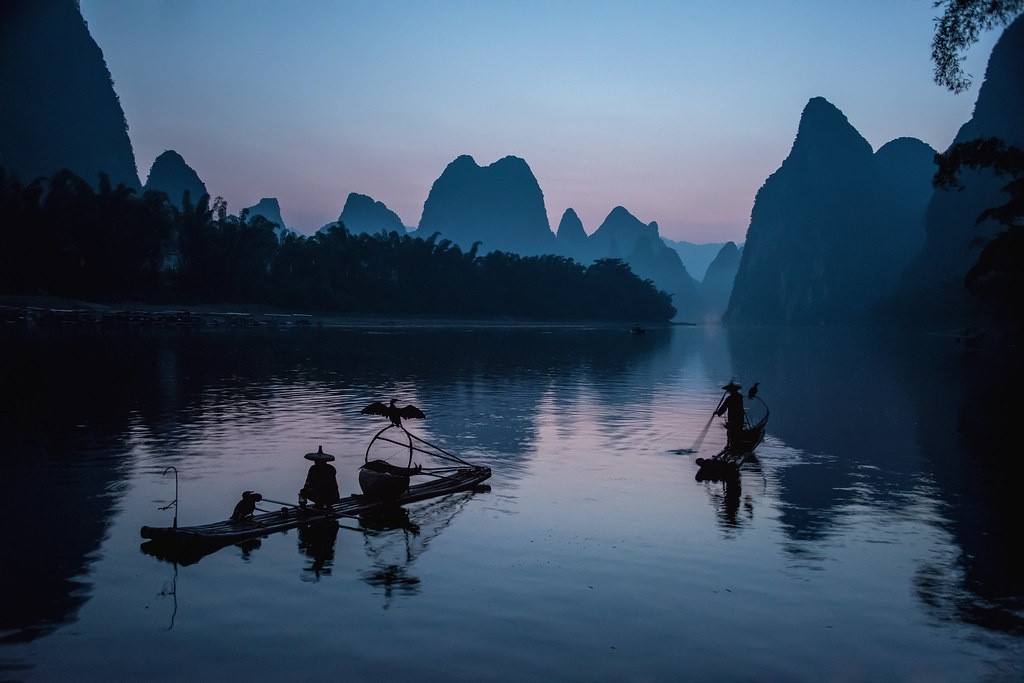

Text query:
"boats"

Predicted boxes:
[[141, 393, 492, 542], [695, 413, 770, 483]]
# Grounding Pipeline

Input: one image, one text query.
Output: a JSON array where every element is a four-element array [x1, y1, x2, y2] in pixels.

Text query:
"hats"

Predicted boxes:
[[304, 446, 335, 461], [721, 383, 740, 390]]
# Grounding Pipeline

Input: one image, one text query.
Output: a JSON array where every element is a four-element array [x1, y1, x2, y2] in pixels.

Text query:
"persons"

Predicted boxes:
[[300, 445, 340, 509], [716, 381, 745, 449]]
